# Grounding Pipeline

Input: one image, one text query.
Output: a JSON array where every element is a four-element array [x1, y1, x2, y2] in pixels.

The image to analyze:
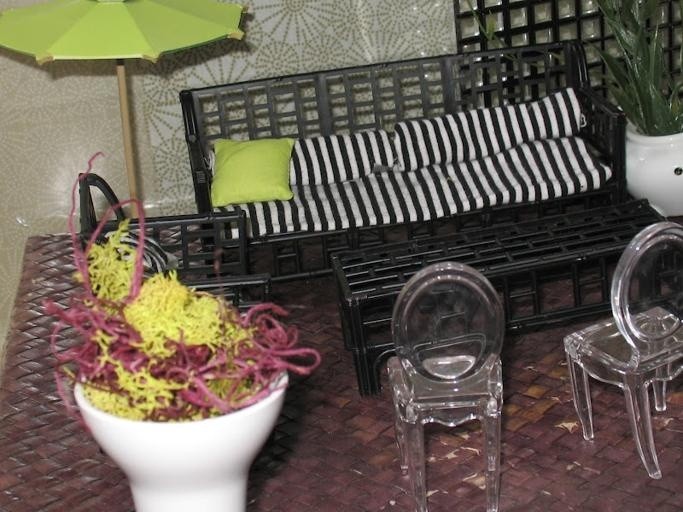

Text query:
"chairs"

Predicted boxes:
[[386, 261, 505, 512], [78, 171, 270, 316], [564, 222, 683, 478]]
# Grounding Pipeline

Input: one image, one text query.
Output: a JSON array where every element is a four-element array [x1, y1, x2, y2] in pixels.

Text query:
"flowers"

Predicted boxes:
[[42, 174, 321, 424]]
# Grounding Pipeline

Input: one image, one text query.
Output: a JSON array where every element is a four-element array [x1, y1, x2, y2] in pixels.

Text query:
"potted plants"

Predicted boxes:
[[587, 0, 683, 217]]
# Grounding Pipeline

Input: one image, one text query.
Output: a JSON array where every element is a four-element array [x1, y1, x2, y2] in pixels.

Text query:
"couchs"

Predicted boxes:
[[180, 39, 628, 296]]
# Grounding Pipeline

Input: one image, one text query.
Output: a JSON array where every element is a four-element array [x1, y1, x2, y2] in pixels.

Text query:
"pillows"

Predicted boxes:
[[209, 136, 297, 207]]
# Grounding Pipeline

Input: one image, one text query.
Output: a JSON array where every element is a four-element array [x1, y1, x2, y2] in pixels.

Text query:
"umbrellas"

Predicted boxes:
[[0, 0, 250, 218]]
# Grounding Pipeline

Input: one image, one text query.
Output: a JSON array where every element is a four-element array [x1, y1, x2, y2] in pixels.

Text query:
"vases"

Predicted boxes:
[[72, 369, 291, 510]]
